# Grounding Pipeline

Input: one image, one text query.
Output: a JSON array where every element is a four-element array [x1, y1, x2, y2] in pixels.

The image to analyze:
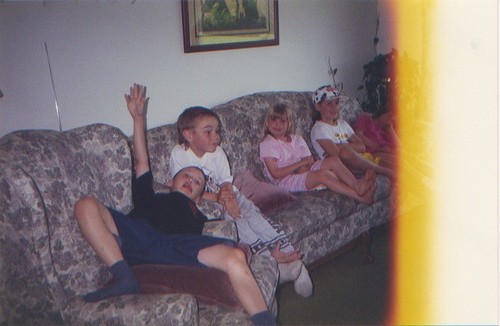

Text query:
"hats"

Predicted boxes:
[[311, 85, 349, 105]]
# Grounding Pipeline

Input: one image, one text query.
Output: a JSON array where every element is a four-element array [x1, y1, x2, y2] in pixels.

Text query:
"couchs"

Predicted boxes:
[[126, 90, 423, 287], [0, 124, 282, 326]]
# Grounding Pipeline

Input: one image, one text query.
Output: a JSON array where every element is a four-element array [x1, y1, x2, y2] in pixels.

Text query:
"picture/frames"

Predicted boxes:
[[181, 0, 280, 53]]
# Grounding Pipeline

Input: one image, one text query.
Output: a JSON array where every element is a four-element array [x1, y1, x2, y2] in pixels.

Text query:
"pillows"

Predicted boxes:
[[232, 169, 300, 212], [131, 261, 241, 312]]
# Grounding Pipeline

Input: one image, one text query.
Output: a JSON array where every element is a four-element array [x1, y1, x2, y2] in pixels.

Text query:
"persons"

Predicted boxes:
[[260, 104, 377, 205], [353, 109, 399, 156], [310, 85, 392, 176], [74, 82, 277, 326], [169, 106, 313, 298]]
